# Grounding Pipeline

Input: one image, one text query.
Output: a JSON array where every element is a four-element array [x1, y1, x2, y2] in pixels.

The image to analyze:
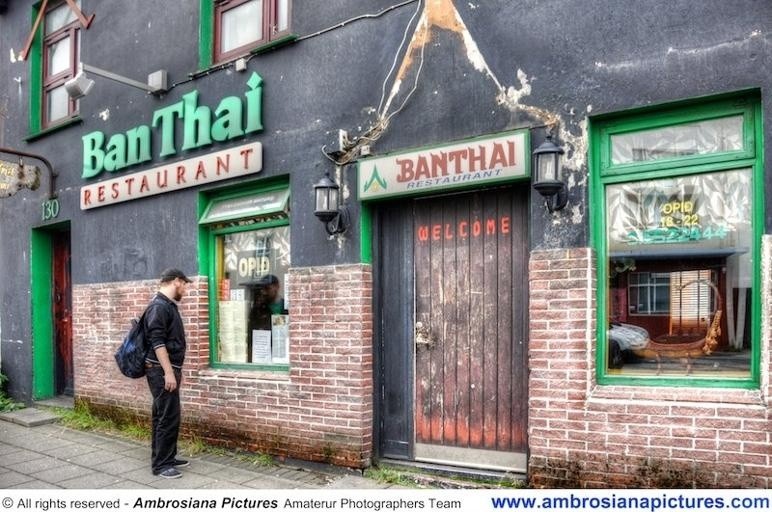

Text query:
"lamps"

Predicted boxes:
[[65, 63, 167, 101], [532, 135, 568, 214], [313, 170, 350, 236]]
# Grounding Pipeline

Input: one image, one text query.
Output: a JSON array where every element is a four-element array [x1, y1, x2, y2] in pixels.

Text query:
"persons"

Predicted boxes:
[[115, 267, 194, 478], [248, 275, 289, 346]]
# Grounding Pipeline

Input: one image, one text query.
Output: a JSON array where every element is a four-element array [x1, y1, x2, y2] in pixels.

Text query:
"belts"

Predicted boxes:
[[145, 363, 161, 368]]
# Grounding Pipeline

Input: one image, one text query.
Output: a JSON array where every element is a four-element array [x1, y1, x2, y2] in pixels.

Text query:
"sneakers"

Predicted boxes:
[[160, 468, 183, 479], [172, 460, 190, 468]]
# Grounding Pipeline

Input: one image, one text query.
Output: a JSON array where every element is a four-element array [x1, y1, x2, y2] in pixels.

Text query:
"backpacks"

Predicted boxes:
[[115, 301, 173, 378]]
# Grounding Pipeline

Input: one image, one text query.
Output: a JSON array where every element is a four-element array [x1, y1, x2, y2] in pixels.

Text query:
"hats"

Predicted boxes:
[[255, 275, 278, 286], [161, 268, 191, 283]]
